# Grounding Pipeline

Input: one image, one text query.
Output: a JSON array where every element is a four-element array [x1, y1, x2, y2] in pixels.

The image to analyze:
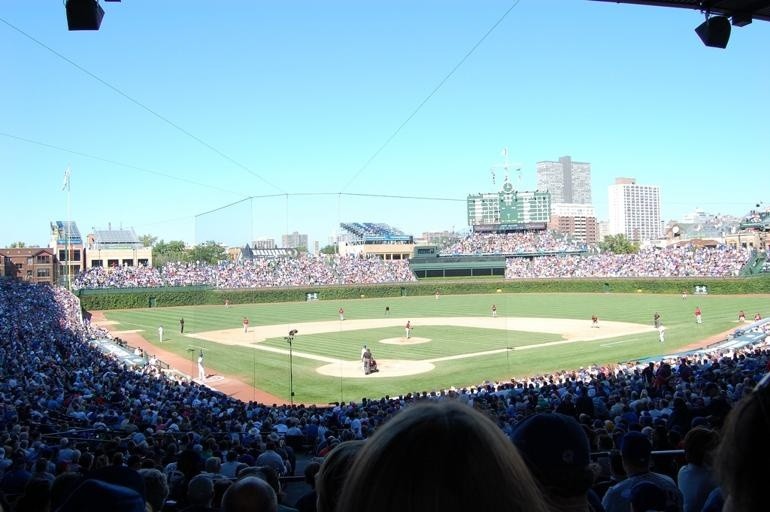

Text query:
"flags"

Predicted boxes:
[[61, 166, 71, 190]]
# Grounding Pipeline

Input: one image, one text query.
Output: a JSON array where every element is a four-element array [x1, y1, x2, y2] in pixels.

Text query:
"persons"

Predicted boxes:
[[754, 312, 761, 321], [438, 229, 584, 255], [224, 298, 230, 310], [242, 315, 249, 333], [288, 328, 298, 340], [591, 314, 600, 329], [751, 322, 770, 345], [737, 200, 769, 235], [504, 238, 770, 281], [491, 304, 497, 317], [0, 274, 175, 512], [654, 311, 663, 329], [405, 320, 412, 340], [682, 288, 688, 300], [338, 307, 344, 321], [738, 310, 745, 322], [339, 221, 412, 246], [74, 248, 420, 293], [435, 291, 440, 300], [657, 325, 664, 342], [383, 306, 391, 316], [180, 317, 184, 334], [694, 305, 703, 324], [176, 344, 770, 512]]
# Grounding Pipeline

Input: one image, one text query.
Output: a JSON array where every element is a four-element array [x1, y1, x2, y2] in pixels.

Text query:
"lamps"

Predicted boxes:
[[693, 0, 737, 49], [64, 0, 105, 30]]
